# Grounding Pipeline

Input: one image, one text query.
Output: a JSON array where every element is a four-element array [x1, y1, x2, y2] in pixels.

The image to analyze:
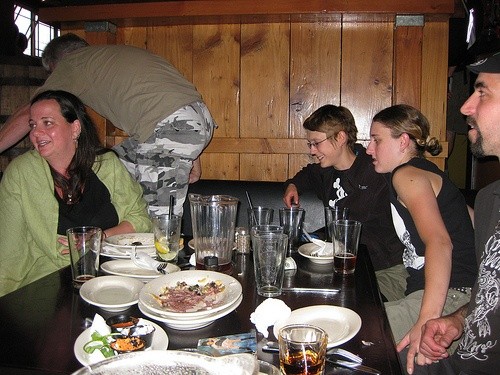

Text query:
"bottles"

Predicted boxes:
[[235, 227, 250, 254], [204, 256, 218, 272]]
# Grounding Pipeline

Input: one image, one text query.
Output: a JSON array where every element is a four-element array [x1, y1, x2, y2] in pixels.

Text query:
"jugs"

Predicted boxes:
[[188, 193, 240, 276]]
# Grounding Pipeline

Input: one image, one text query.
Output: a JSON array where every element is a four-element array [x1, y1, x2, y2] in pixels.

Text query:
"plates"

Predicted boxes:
[[138, 270, 243, 331], [71, 349, 250, 375], [188, 238, 238, 251], [73, 318, 169, 366], [100, 259, 181, 284], [272, 305, 362, 349], [89, 233, 167, 260]]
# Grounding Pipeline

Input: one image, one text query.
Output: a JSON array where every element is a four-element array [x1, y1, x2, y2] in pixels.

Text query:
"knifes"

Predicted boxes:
[[262, 345, 381, 375]]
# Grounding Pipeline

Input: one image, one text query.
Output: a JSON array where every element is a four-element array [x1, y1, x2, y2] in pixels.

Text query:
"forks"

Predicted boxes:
[[266, 342, 363, 364]]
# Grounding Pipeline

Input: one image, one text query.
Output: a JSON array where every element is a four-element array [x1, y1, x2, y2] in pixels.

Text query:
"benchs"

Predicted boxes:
[[180, 179, 325, 236]]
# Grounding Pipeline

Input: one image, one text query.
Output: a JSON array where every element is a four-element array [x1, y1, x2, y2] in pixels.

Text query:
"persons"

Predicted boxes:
[[397, 56, 500, 374], [282, 104, 408, 302], [367, 104, 477, 343], [0, 32, 214, 233], [0, 91, 153, 298]]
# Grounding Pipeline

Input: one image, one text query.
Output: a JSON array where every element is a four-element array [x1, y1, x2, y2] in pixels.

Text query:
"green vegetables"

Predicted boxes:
[[82, 332, 123, 358]]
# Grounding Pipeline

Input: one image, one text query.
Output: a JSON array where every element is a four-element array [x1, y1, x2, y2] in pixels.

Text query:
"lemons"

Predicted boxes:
[[155, 242, 169, 254]]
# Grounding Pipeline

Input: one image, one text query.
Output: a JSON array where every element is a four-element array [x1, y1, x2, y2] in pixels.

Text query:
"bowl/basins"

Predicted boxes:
[[298, 242, 342, 264], [79, 275, 144, 312]]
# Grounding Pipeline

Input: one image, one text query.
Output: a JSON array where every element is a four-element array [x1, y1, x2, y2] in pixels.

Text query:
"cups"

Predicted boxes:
[[248, 208, 305, 298], [66, 226, 102, 289], [326, 206, 349, 242], [277, 323, 328, 375], [151, 213, 182, 265], [332, 220, 361, 275]]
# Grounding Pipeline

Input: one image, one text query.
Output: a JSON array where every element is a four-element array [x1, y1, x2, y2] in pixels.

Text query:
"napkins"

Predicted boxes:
[[249, 298, 291, 340]]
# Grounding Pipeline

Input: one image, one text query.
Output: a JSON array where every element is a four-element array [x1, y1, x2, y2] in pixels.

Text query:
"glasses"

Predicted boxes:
[[306, 134, 334, 148]]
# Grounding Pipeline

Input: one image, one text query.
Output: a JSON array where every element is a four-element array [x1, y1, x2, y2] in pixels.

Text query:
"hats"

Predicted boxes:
[[466, 53, 500, 73]]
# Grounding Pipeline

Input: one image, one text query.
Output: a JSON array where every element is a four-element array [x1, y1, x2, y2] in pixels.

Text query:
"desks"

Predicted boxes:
[[0, 236, 405, 375]]
